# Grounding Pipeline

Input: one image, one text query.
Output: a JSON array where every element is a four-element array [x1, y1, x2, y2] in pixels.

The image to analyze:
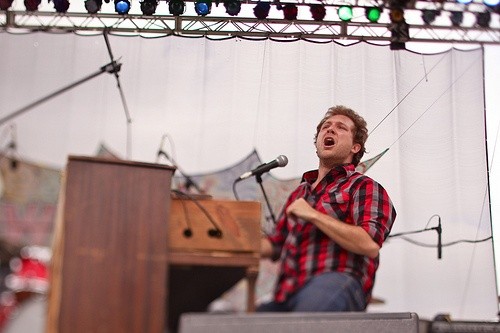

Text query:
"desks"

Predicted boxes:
[[45, 155, 177, 333]]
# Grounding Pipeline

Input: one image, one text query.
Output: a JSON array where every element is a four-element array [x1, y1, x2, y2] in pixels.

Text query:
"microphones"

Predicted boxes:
[[438, 217, 442, 258], [236, 154, 288, 183]]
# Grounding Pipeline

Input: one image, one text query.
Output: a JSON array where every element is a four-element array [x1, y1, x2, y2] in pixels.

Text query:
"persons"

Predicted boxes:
[[258, 105, 398, 313]]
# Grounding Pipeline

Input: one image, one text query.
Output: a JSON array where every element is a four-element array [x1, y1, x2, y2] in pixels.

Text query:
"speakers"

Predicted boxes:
[[177, 312, 420, 333]]
